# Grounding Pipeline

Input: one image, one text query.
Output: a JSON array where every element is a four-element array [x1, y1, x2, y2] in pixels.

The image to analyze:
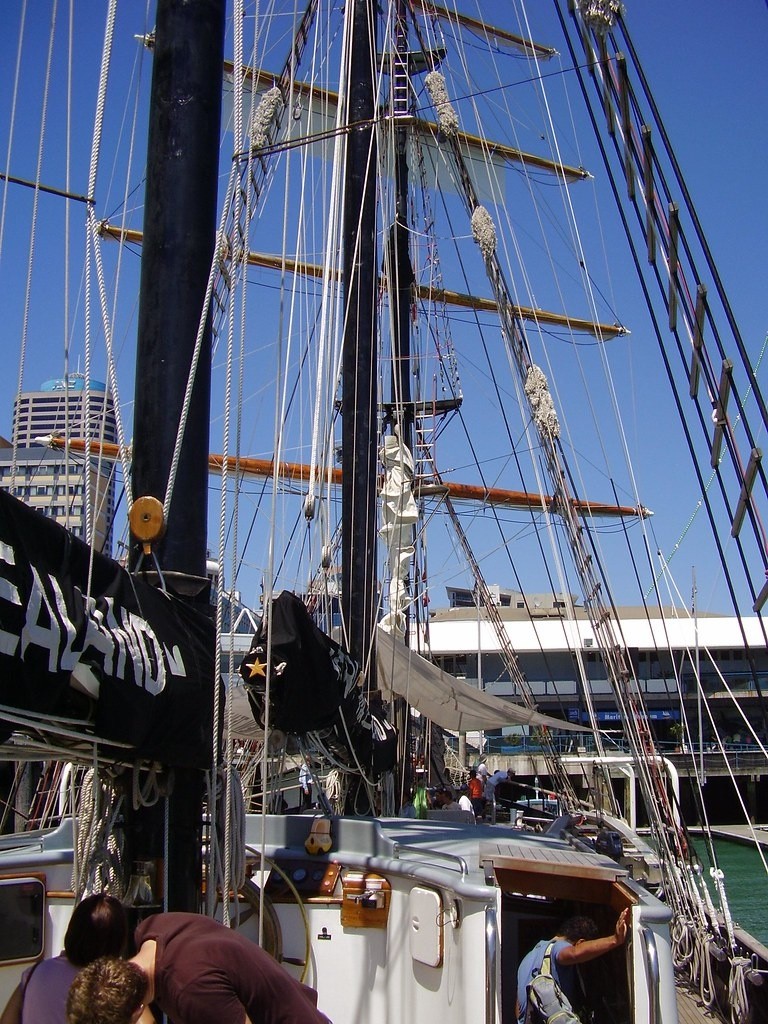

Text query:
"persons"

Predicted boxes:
[[297, 753, 518, 823], [0, 894, 334, 1024], [515, 907, 630, 1024], [699, 730, 752, 750]]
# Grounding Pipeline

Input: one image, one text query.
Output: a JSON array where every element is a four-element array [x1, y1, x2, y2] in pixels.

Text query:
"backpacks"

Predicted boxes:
[[522, 945, 586, 1023]]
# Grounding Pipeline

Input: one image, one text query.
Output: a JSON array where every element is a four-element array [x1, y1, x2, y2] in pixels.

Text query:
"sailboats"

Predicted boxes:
[[0, 0, 767, 1024]]
